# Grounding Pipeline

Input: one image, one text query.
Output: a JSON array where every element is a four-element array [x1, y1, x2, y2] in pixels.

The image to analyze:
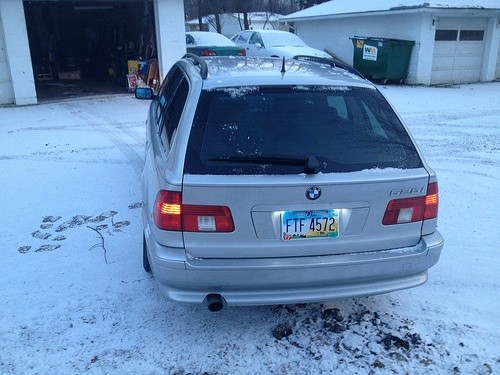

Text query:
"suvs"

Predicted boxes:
[[136, 53, 445, 313]]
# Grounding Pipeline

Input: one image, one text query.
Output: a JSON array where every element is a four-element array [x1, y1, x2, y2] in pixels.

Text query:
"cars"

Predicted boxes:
[[230, 30, 333, 66], [186, 31, 246, 56]]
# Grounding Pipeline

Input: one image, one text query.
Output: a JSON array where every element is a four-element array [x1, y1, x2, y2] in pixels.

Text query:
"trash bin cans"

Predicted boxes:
[[349, 36, 415, 85]]
